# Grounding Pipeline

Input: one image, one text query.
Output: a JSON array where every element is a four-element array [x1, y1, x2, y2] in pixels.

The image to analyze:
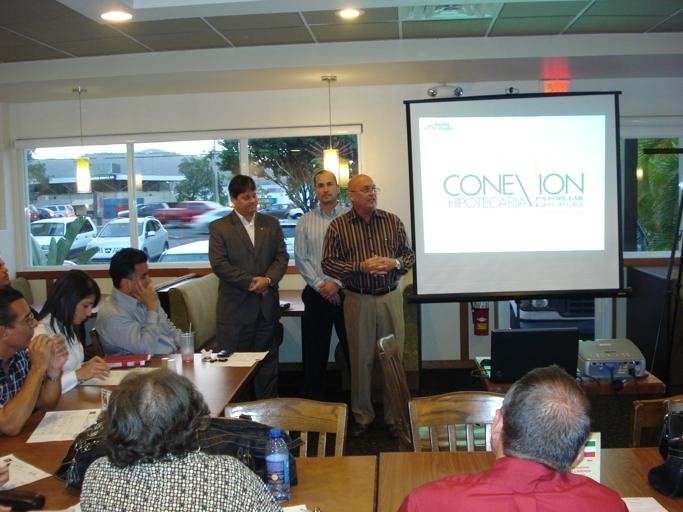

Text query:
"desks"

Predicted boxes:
[[276, 287, 305, 377]]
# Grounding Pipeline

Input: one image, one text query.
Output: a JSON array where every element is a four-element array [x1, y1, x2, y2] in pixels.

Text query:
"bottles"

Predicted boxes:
[[264, 428, 290, 500]]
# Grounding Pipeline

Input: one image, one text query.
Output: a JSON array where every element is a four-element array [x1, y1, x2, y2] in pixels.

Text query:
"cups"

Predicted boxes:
[[178, 332, 195, 367]]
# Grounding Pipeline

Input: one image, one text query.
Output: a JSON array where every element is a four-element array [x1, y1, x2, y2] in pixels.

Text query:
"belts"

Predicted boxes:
[[348, 285, 396, 295]]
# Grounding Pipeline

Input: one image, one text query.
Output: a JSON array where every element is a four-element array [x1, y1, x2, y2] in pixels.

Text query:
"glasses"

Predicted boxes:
[[351, 188, 377, 192]]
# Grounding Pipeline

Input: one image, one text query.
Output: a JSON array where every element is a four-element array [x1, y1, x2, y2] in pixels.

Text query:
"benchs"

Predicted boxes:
[[151, 272, 285, 371]]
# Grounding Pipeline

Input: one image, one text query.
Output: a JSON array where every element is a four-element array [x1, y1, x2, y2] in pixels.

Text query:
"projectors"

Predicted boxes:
[[577, 337, 646, 380]]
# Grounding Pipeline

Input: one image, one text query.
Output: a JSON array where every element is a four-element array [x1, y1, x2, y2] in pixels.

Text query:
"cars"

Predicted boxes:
[[28, 196, 235, 231], [259, 197, 308, 261], [28, 215, 96, 259], [158, 235, 213, 263], [87, 216, 167, 262]]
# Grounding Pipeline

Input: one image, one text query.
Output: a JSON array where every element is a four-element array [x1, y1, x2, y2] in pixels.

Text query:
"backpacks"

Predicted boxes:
[[55, 415, 304, 490]]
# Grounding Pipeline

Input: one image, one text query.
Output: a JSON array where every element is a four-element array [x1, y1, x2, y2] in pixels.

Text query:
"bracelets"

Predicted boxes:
[[394, 260, 401, 269], [45, 370, 64, 381]]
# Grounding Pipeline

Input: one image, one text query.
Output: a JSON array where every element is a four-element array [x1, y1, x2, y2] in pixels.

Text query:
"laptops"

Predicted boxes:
[[474, 327, 580, 384]]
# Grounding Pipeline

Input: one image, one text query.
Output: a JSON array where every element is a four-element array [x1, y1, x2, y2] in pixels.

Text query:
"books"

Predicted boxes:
[[104, 352, 152, 369]]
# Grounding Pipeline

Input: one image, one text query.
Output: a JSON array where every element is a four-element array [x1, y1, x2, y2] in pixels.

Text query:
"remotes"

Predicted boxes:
[[0, 488, 45, 509]]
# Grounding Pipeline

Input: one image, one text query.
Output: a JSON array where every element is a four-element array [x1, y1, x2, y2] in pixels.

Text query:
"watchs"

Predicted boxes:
[[266, 277, 273, 288]]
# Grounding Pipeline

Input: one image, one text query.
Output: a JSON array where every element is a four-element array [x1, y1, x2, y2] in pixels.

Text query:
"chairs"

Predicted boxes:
[[377, 334, 496, 452]]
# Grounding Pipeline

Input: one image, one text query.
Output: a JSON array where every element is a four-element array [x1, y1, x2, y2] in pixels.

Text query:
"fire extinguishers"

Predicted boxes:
[[470, 299, 489, 336]]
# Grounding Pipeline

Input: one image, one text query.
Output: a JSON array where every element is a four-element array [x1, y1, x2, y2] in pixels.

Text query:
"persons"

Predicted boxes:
[[323, 173, 417, 440], [0, 286, 68, 437], [397, 364, 627, 512], [95, 248, 185, 356], [29, 268, 110, 394], [206, 173, 291, 401], [0, 460, 13, 512], [0, 259, 11, 287], [78, 366, 281, 512], [293, 171, 356, 404]]
